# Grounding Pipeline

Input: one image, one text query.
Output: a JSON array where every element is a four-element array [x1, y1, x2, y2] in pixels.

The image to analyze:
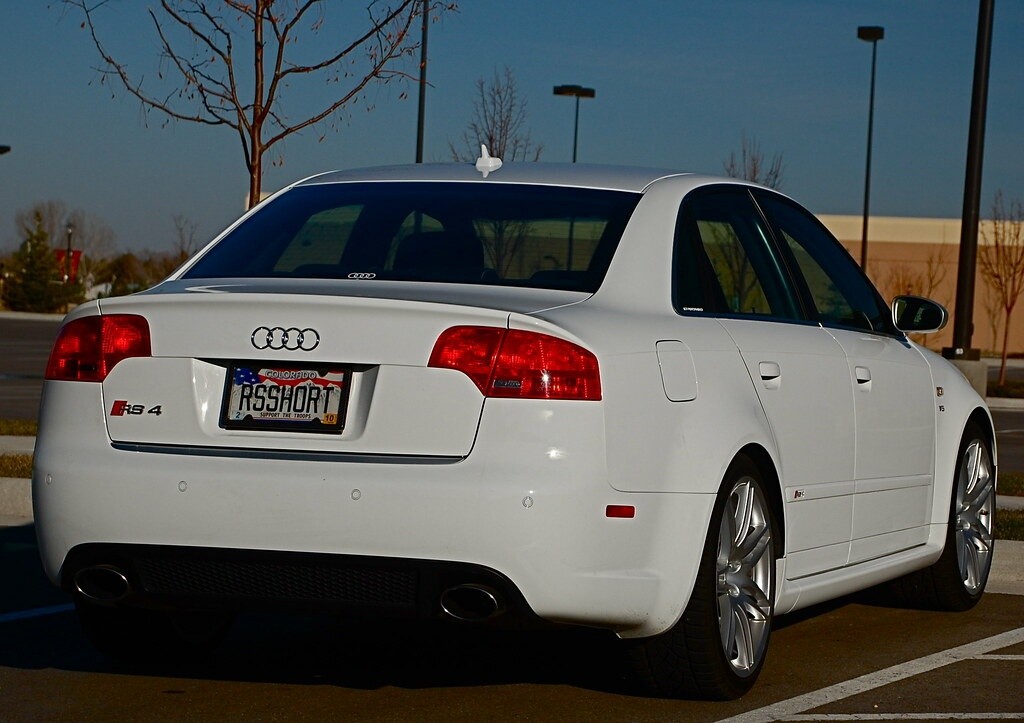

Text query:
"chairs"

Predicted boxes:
[[393, 230, 485, 284]]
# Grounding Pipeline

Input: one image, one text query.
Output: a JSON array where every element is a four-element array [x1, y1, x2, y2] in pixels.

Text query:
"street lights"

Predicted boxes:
[[552, 84, 597, 271], [858, 25, 886, 276], [63, 220, 74, 317]]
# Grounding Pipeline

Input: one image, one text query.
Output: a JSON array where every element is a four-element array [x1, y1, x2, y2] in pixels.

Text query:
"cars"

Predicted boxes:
[[31, 159, 1000, 702]]
